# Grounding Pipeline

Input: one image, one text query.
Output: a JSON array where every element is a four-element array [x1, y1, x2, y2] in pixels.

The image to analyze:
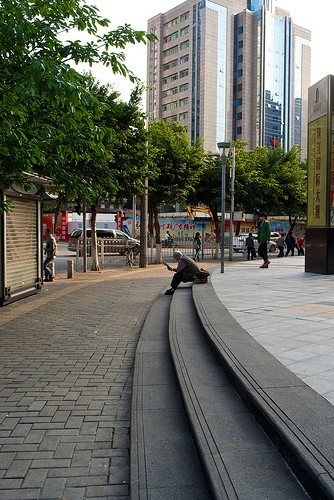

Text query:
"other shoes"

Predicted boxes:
[[264, 261, 270, 266], [260, 265, 268, 268], [164, 288, 175, 295], [44, 276, 54, 282]]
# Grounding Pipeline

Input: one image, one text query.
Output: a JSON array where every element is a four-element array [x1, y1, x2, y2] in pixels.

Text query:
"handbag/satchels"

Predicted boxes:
[[193, 268, 210, 284], [252, 252, 256, 257]]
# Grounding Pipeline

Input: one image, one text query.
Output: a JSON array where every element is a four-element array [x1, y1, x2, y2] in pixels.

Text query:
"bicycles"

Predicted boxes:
[[125, 245, 136, 267]]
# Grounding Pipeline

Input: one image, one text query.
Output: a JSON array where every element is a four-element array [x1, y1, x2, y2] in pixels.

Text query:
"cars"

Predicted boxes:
[[270, 232, 282, 242], [67, 228, 141, 255], [232, 234, 277, 253]]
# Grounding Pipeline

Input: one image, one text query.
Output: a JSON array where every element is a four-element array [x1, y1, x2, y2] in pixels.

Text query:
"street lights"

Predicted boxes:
[[216, 142, 231, 273]]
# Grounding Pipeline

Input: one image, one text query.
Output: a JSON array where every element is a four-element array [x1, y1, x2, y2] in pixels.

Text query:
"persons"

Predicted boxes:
[[285, 231, 296, 257], [258, 212, 270, 268], [245, 233, 255, 260], [277, 233, 286, 258], [44, 228, 57, 282], [164, 231, 172, 248], [164, 251, 201, 295], [295, 237, 305, 256], [193, 232, 202, 262]]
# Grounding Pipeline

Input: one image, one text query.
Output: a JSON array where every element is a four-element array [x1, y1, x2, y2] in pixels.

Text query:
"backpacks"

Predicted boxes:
[[193, 239, 199, 249]]
